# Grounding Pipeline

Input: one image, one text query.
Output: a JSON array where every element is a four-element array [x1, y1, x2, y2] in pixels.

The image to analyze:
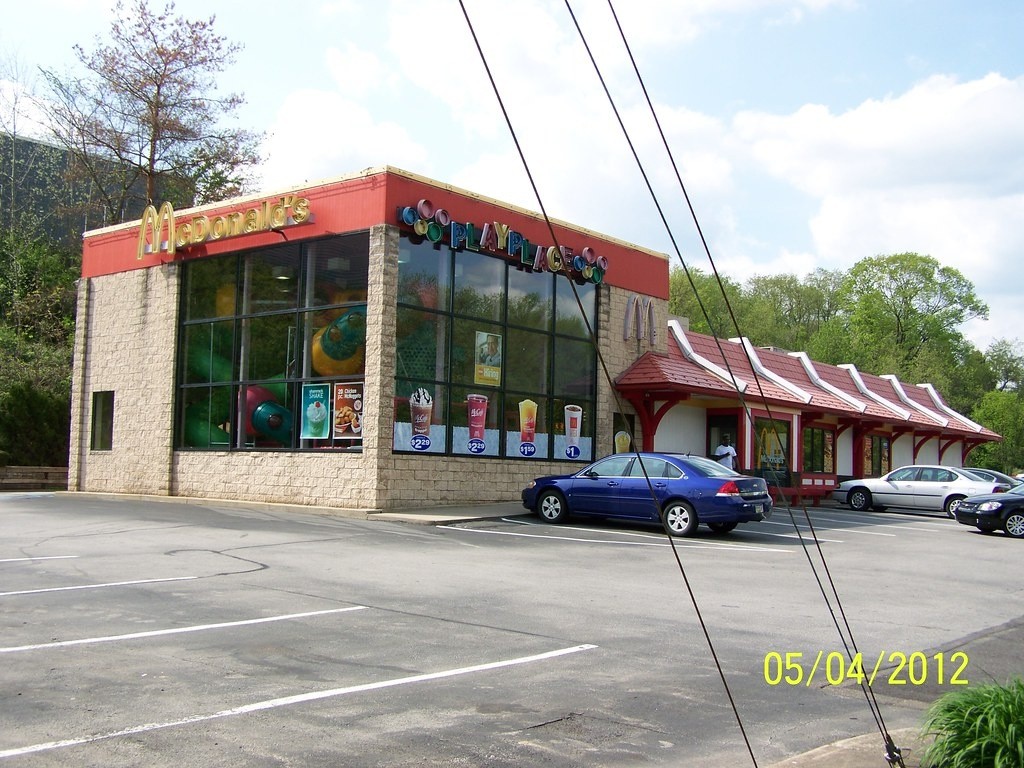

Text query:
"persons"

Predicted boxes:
[[484, 334, 501, 368], [713, 434, 741, 474]]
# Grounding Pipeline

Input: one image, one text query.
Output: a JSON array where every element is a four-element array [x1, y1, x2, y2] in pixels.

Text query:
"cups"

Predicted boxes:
[[409, 399, 433, 439], [306, 409, 327, 438], [519, 401, 538, 442], [564, 405, 582, 448], [615, 431, 631, 455], [468, 393, 488, 439]]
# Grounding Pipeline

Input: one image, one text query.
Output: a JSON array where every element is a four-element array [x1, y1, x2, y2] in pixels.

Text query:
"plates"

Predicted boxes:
[[336, 413, 356, 427]]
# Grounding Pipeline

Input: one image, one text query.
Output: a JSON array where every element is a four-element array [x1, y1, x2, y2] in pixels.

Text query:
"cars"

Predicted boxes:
[[521, 453, 773, 537], [938, 467, 1024, 490], [833, 464, 1011, 520], [763, 466, 788, 485], [954, 482, 1024, 537]]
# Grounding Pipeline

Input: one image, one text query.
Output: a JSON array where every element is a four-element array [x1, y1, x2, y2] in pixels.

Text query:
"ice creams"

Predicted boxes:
[[409, 387, 433, 437], [306, 401, 327, 437]]
